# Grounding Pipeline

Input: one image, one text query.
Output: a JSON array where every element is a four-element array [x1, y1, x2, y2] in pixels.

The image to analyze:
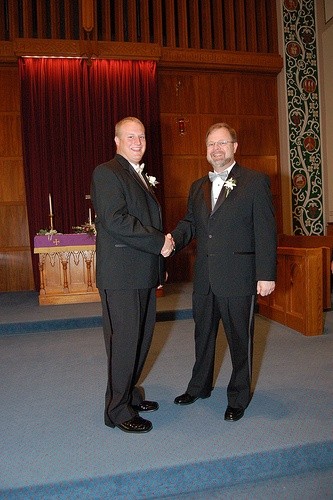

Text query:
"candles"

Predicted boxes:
[[49, 194, 53, 215]]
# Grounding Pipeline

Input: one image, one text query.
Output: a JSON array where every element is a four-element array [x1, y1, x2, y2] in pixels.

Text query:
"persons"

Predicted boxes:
[[161, 123, 278, 421], [91, 117, 172, 433]]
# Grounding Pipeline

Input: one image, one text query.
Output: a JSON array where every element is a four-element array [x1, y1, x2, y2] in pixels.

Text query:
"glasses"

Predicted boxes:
[[206, 140, 235, 147]]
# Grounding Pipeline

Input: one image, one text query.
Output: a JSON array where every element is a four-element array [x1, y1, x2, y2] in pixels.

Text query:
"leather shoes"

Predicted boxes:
[[174, 392, 211, 406], [224, 406, 245, 422], [133, 401, 159, 412], [105, 414, 153, 433]]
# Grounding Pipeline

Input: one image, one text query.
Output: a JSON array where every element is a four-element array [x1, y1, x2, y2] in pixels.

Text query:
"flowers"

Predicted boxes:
[[224, 178, 237, 197], [146, 173, 158, 187]]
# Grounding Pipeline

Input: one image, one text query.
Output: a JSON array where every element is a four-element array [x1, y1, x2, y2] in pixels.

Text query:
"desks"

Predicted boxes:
[[33, 232, 167, 307]]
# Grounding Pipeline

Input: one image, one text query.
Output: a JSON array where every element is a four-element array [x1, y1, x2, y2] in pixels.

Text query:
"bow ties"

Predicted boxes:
[[209, 170, 228, 182], [136, 163, 145, 174]]
